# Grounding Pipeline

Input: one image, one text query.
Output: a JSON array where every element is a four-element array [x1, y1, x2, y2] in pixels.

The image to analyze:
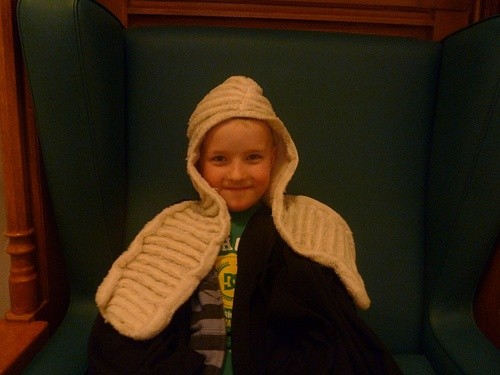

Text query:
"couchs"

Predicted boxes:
[[1, 1, 500, 375]]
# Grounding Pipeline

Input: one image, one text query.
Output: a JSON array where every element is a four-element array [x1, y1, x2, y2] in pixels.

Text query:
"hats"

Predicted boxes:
[[94, 74, 371, 343]]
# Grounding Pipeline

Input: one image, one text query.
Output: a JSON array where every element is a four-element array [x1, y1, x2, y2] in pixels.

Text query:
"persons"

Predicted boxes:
[[82, 73, 405, 374]]
